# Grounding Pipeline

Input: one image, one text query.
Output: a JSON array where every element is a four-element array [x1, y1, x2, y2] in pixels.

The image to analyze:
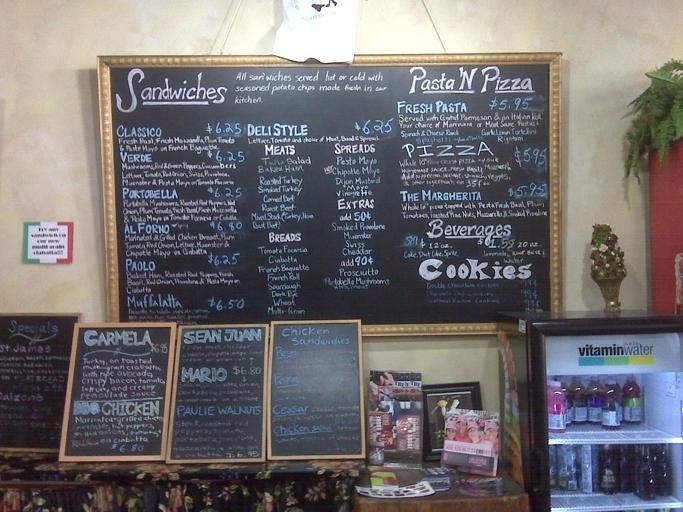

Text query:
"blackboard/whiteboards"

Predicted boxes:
[[0, 313, 83, 453], [165, 322, 269, 466], [59, 321, 178, 464], [265, 318, 368, 465], [97, 53, 565, 337]]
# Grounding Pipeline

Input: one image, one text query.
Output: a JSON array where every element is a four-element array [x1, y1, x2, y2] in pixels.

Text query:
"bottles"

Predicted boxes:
[[547, 377, 673, 501]]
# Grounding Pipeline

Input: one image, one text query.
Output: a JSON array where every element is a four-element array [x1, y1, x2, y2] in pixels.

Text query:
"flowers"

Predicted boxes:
[[587, 219, 625, 282]]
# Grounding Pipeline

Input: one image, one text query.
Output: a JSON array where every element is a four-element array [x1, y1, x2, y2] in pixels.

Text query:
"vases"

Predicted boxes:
[[595, 275, 624, 310]]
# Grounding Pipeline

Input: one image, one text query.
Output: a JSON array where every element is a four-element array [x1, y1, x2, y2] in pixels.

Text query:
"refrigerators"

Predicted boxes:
[[496, 310, 682, 512]]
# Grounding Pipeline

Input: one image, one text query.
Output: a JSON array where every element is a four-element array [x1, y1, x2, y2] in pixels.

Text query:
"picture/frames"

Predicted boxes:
[[419, 381, 484, 466]]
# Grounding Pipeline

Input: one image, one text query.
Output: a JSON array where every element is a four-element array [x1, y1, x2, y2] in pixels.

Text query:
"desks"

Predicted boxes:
[[346, 466, 530, 510]]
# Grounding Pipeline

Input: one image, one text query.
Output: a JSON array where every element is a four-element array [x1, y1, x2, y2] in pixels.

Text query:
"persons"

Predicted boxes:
[[444, 413, 500, 458], [368, 372, 396, 412]]
